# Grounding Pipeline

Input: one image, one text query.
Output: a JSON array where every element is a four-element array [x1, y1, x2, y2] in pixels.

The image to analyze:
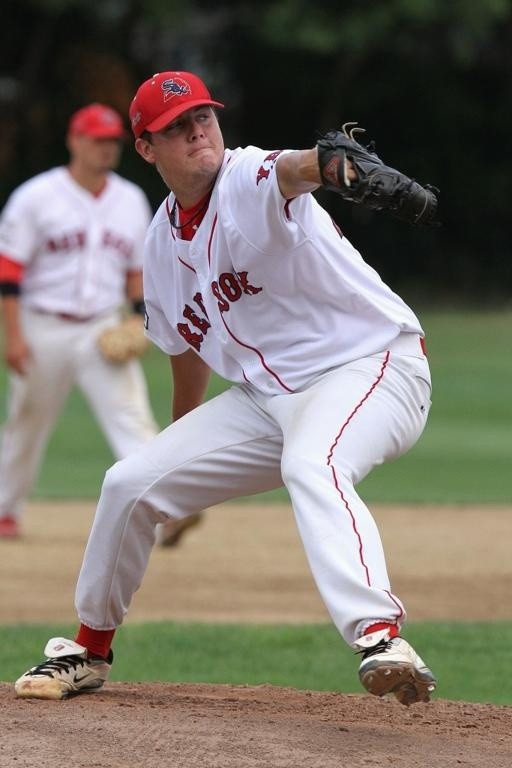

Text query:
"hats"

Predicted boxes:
[[128, 68, 224, 139], [69, 101, 127, 142]]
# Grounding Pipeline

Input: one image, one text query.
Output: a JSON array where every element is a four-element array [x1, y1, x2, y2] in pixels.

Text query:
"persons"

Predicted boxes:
[[14, 71, 447, 709], [1, 106, 206, 548]]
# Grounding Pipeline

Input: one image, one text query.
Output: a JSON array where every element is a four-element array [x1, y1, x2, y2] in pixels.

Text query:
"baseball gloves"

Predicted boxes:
[[96, 313, 148, 366], [317, 127, 441, 226]]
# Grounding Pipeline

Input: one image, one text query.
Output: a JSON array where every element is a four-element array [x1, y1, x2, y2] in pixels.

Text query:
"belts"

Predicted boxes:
[[26, 306, 120, 323]]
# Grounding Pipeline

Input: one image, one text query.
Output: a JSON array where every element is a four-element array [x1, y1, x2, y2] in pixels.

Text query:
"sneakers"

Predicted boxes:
[[14, 637, 114, 701], [0, 516, 23, 540], [350, 626, 436, 707], [156, 510, 202, 547]]
